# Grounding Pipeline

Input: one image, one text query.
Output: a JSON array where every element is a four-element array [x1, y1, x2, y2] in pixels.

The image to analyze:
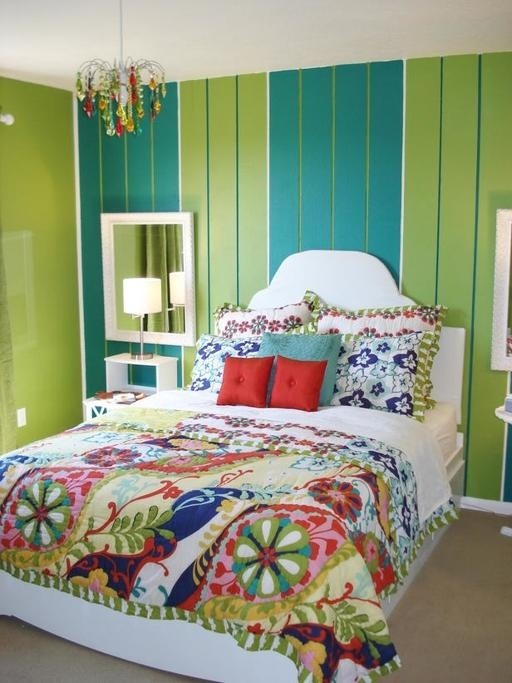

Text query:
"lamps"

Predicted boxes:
[[123, 277, 162, 361], [167, 271, 185, 311], [76, 0, 167, 137]]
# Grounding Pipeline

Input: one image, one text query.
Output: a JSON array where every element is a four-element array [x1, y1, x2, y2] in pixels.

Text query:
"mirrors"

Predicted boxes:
[[490, 209, 512, 371], [100, 212, 197, 347]]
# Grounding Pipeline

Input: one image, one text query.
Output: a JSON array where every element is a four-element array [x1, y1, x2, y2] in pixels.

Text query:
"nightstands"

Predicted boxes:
[[82, 353, 178, 422]]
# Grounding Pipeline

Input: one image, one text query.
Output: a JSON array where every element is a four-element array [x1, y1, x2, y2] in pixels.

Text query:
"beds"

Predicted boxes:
[[0, 250, 466, 683]]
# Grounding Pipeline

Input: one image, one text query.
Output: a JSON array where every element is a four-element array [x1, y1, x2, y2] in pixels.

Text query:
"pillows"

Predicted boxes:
[[183, 290, 448, 424]]
